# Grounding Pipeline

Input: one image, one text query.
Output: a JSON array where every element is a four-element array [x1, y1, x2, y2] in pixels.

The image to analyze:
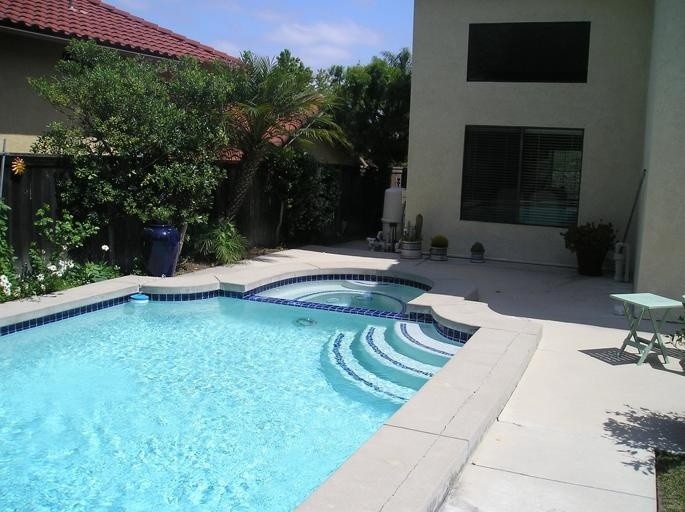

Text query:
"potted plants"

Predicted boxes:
[[470, 241, 487, 263], [428, 233, 449, 260], [401, 214, 423, 258]]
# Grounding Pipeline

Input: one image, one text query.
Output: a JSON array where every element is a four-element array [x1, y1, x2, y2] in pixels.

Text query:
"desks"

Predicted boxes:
[[610, 292, 684, 367]]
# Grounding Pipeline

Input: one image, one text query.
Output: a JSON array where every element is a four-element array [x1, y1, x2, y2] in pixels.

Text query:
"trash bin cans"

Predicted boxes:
[[610, 241, 631, 282]]
